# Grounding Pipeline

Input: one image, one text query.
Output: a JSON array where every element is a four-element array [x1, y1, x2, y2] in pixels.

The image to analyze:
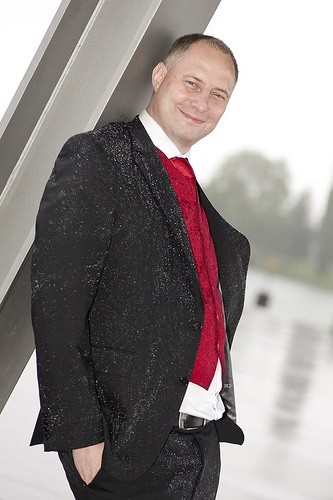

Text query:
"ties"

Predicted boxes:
[[169, 157, 227, 386]]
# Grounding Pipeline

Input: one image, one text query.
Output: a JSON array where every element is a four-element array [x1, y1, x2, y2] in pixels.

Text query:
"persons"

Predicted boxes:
[[29, 34, 252, 500]]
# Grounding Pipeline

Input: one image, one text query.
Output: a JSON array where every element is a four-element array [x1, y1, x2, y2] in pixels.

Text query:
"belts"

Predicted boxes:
[[173, 412, 210, 431]]
